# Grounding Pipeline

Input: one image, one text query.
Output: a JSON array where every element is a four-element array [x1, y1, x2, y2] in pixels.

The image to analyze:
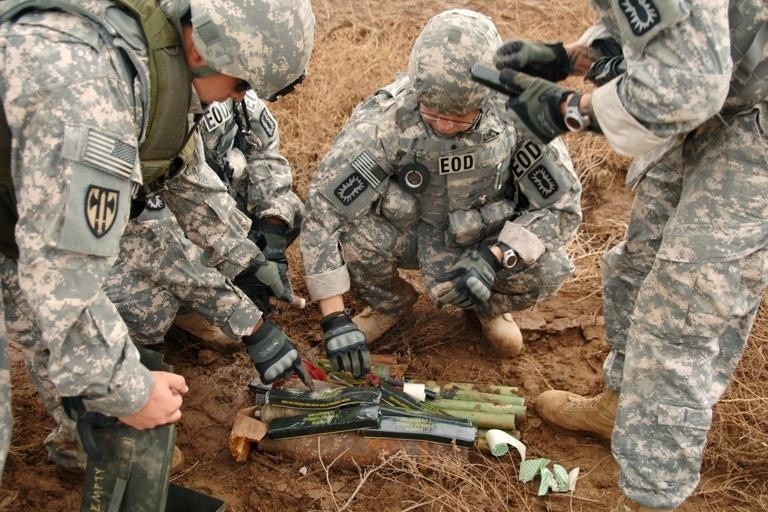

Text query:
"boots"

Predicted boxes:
[[535, 385, 619, 444]]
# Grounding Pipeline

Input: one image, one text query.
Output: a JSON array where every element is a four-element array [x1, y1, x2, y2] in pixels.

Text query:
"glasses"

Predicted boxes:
[[416, 105, 481, 128], [234, 79, 249, 94]]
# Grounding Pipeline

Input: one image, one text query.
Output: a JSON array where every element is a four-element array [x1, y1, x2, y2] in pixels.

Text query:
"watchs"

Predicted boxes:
[[564, 94, 591, 135], [494, 241, 518, 271]]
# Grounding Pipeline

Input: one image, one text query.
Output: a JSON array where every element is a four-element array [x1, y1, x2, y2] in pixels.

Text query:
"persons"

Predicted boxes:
[[1, 0, 312, 510], [299, 9, 581, 382], [494, 0, 768, 512]]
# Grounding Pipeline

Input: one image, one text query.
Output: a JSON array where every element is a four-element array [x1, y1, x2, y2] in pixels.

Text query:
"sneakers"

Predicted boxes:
[[350, 307, 399, 341], [59, 443, 185, 482], [476, 302, 522, 358], [176, 307, 240, 351]]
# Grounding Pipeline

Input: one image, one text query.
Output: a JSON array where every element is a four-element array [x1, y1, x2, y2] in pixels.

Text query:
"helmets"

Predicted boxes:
[[190, 1, 314, 103], [407, 9, 505, 114]]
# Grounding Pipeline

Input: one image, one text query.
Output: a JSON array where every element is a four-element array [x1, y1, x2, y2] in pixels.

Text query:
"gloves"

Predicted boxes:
[[436, 248, 504, 308], [318, 310, 371, 380], [254, 223, 289, 274], [500, 68, 574, 145], [493, 39, 569, 82], [236, 253, 294, 310], [242, 315, 314, 392]]
